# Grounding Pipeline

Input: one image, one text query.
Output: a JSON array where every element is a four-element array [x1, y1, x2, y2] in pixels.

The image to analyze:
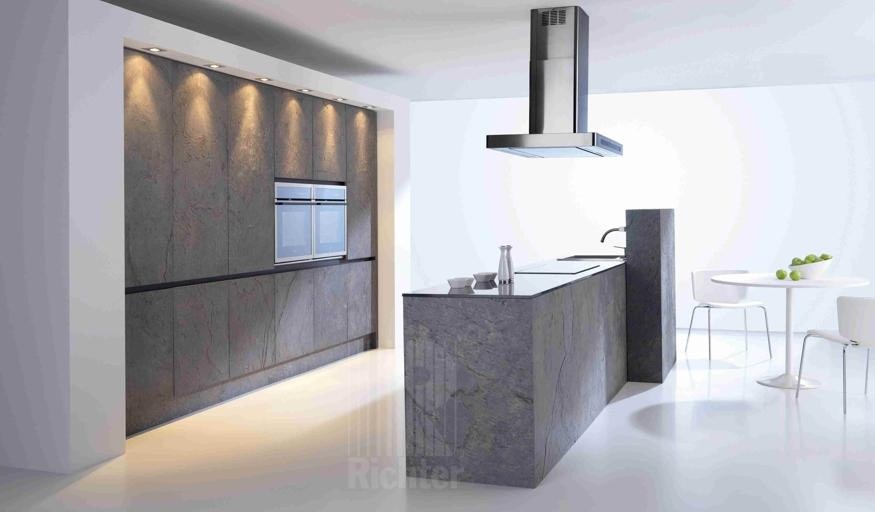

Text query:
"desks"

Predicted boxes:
[[711, 272, 871, 389]]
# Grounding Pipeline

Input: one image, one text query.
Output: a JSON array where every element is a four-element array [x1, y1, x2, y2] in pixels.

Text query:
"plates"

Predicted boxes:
[[788, 256, 834, 279]]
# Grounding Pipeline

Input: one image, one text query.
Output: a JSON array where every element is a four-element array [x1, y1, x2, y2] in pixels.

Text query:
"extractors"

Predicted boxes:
[[485, 5, 624, 160]]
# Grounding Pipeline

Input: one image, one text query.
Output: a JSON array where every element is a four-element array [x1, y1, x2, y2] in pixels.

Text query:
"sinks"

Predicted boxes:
[[557, 255, 620, 260]]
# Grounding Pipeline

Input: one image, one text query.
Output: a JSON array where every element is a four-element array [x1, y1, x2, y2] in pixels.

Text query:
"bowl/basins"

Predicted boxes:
[[448, 288, 476, 295], [447, 276, 475, 290], [473, 271, 497, 282], [473, 281, 498, 291]]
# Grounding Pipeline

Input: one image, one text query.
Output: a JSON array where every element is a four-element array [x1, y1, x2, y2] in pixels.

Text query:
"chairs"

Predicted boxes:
[[795, 295, 875, 414], [684, 269, 773, 361]]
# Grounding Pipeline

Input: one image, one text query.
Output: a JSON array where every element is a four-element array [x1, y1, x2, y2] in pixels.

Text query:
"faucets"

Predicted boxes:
[[601, 226, 626, 243]]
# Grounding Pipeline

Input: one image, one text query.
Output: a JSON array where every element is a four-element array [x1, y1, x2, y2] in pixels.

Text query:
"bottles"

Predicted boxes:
[[497, 244, 509, 284], [506, 244, 512, 282]]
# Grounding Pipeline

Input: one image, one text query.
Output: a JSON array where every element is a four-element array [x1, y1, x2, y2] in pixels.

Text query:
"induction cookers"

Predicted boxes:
[[513, 260, 601, 274]]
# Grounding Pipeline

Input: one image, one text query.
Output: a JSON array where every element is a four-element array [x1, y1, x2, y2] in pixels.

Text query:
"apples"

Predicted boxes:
[[776, 269, 787, 279], [791, 254, 831, 265], [789, 269, 801, 281]]
